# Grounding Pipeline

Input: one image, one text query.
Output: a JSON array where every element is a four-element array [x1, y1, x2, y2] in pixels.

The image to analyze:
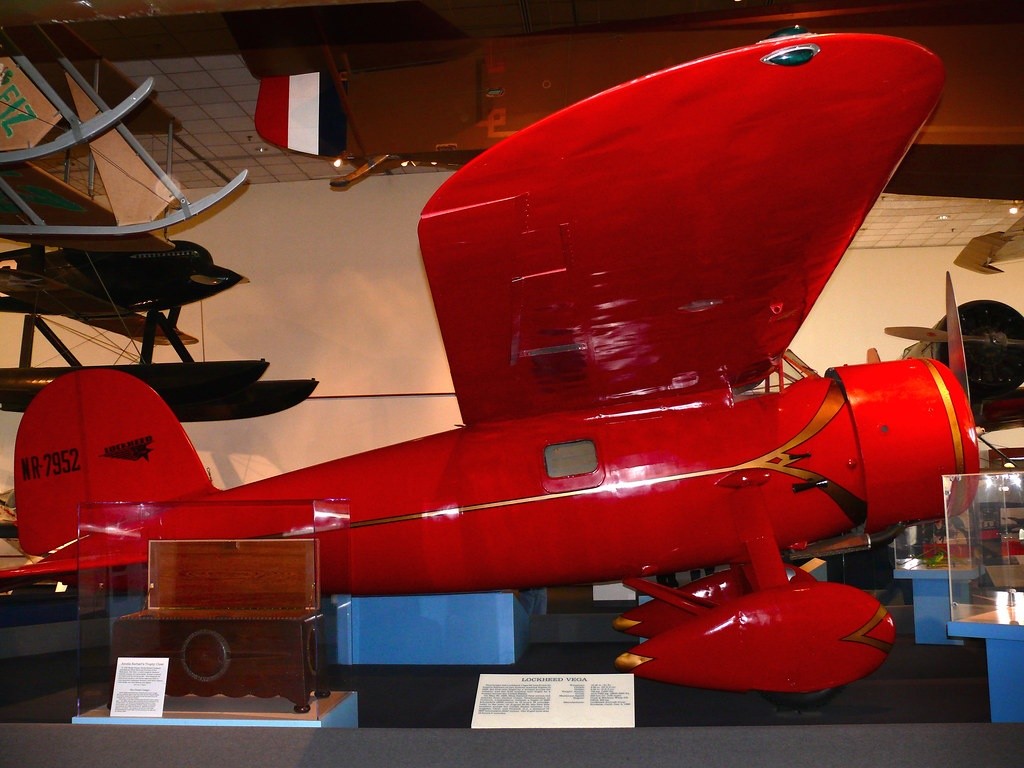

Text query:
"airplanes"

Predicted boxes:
[[0, 29, 1024, 714]]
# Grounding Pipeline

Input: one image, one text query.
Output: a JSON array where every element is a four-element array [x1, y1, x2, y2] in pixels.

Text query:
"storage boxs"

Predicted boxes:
[[109, 538, 338, 715]]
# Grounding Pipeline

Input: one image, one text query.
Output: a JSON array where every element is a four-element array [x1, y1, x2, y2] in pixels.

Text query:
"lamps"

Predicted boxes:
[[1008, 203, 1023, 214]]
